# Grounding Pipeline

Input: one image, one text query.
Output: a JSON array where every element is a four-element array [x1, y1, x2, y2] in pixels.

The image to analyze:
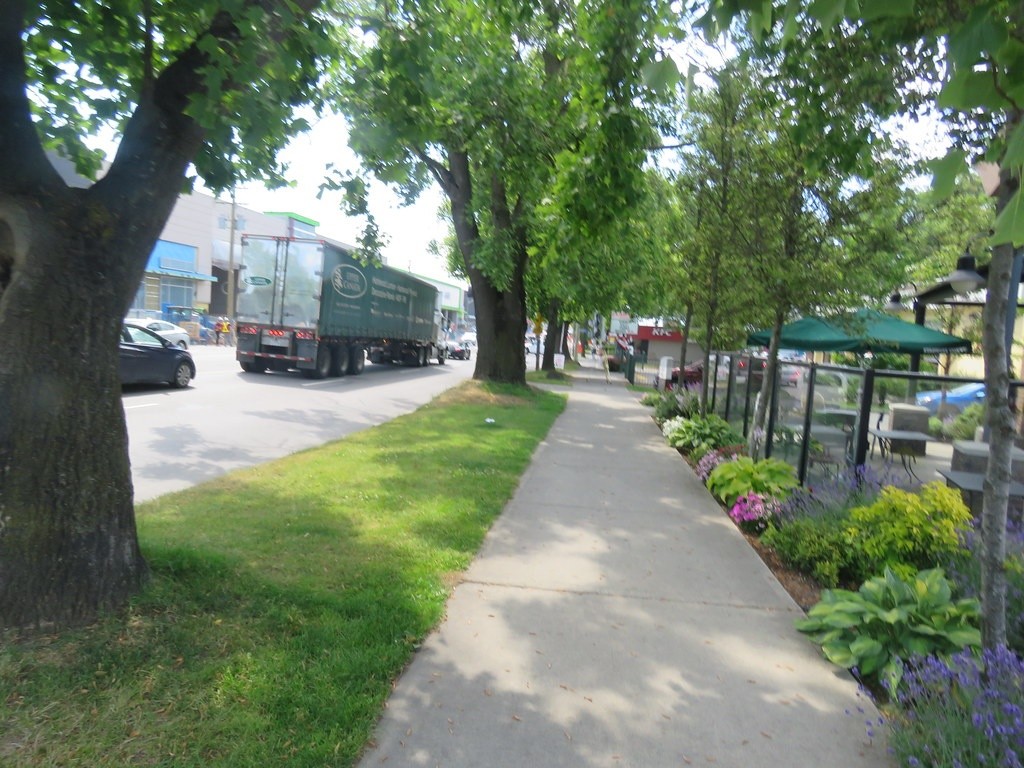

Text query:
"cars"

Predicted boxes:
[[446, 331, 546, 361], [117, 323, 196, 389], [913, 383, 987, 425], [653, 351, 802, 392], [120, 317, 190, 349]]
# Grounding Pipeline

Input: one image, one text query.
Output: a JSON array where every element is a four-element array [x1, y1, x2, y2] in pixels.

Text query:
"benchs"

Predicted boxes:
[[808, 444, 839, 485]]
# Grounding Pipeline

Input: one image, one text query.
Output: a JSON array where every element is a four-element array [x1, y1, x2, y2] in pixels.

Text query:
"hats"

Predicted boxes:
[[218, 317, 223, 320], [224, 317, 229, 321]]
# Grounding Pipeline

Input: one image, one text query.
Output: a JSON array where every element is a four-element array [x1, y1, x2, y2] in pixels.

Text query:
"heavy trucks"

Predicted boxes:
[[236, 232, 450, 378]]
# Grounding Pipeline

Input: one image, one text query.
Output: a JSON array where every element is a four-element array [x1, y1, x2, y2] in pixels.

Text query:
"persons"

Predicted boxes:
[[626, 342, 634, 359], [214, 316, 238, 347]]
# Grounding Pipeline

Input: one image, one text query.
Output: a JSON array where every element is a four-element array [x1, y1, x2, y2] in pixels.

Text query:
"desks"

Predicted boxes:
[[935, 467, 1024, 531], [869, 429, 936, 486]]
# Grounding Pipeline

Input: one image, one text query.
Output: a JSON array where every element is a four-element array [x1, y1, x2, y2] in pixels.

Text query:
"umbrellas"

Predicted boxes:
[[747, 305, 974, 470]]
